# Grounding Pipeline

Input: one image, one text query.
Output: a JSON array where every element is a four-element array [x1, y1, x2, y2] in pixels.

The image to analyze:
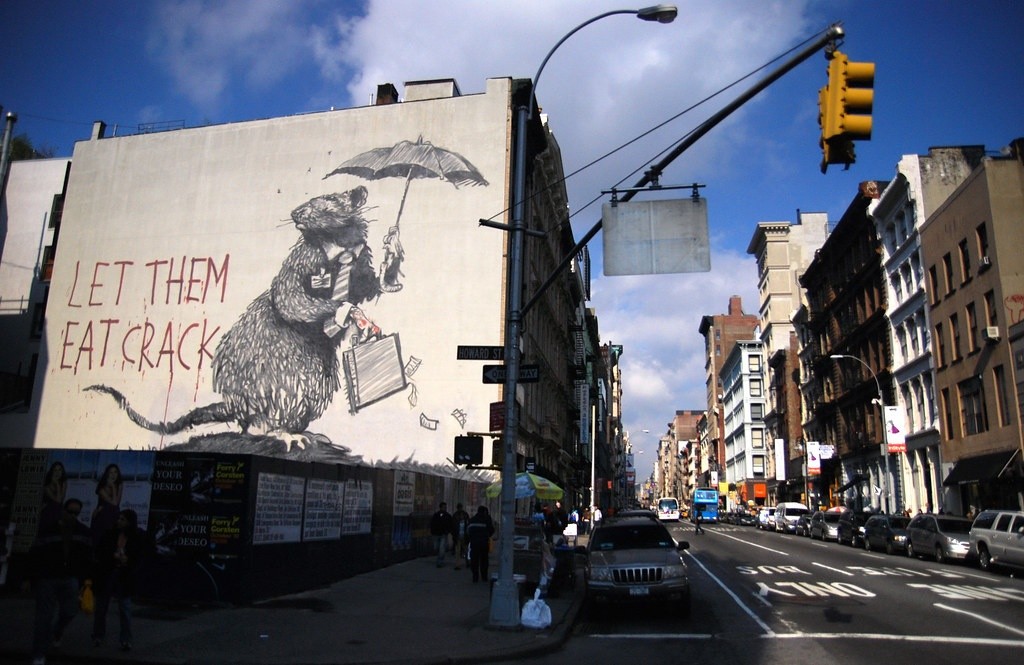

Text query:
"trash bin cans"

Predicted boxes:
[[548, 546, 576, 596]]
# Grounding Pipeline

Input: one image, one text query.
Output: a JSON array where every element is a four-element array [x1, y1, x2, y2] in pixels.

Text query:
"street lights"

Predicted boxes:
[[486, 1, 680, 633], [830, 355, 893, 517], [624, 430, 649, 513]]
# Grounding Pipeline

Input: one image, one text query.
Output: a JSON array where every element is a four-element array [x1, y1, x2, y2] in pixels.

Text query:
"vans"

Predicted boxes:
[[658, 497, 680, 522], [775, 502, 808, 534]]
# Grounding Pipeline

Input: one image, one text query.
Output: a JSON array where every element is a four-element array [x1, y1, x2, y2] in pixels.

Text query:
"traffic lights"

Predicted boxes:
[[453, 435, 484, 466], [828, 51, 877, 145], [818, 85, 858, 172]]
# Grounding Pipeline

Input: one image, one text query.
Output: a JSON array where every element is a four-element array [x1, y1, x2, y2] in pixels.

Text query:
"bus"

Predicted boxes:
[[690, 487, 719, 524]]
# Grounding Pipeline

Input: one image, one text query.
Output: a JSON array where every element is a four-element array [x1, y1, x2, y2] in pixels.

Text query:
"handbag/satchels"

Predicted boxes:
[[489, 537, 494, 553], [521, 589, 552, 628]]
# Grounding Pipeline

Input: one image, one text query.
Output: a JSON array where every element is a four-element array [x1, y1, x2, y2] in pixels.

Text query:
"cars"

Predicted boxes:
[[863, 514, 912, 555], [796, 514, 813, 537], [904, 513, 974, 563], [719, 506, 776, 531]]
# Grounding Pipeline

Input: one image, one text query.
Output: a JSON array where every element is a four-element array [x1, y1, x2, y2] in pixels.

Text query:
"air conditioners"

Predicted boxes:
[[980, 256, 990, 268], [981, 326, 1000, 340]]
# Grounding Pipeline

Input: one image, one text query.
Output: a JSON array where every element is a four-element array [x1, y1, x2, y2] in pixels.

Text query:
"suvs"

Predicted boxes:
[[968, 510, 1024, 573], [580, 516, 690, 616], [810, 511, 845, 541], [838, 510, 881, 549]]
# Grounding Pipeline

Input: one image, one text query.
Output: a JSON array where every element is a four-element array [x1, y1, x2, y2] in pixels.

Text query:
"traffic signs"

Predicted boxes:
[[482, 364, 538, 384]]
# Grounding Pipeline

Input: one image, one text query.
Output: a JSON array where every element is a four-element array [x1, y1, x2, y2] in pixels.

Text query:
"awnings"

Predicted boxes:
[[833, 476, 869, 493], [943, 449, 1017, 486]]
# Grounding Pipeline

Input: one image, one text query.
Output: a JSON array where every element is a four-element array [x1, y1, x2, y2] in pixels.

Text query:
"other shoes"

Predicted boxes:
[[34, 656, 46, 665], [121, 641, 132, 650]]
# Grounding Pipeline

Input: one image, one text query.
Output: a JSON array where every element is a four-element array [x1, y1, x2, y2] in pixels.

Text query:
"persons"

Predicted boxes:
[[39, 461, 68, 510], [90, 463, 123, 546], [431, 501, 495, 583], [530, 501, 607, 544], [695, 509, 705, 535], [24, 497, 145, 665]]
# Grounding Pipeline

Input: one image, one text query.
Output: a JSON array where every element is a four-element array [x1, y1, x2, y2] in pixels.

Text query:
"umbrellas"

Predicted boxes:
[[486, 470, 564, 516]]
